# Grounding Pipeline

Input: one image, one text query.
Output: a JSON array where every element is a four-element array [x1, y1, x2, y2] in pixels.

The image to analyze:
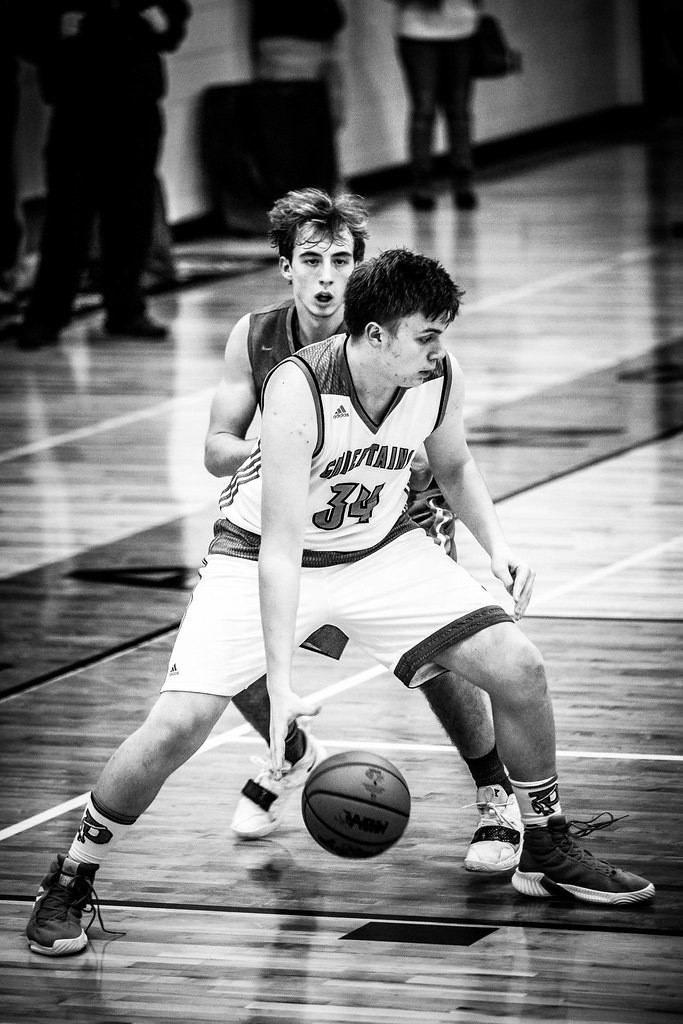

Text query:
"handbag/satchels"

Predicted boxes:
[[472, 11, 516, 78]]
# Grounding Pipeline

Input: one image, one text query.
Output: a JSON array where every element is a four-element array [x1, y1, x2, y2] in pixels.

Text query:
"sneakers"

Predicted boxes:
[[513, 815, 655, 905], [462, 783, 524, 872], [26, 852, 101, 955], [229, 726, 329, 836]]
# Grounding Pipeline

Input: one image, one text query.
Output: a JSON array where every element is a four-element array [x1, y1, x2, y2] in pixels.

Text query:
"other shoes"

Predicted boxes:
[[450, 181, 477, 208], [103, 311, 169, 339], [14, 320, 61, 351], [410, 185, 435, 211]]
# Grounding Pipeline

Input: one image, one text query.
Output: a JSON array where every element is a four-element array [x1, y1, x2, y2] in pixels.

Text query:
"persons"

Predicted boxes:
[[388, 0, 484, 214], [0, 130, 26, 316], [247, 0, 348, 82], [9, 1, 193, 350], [82, 170, 178, 291], [209, 188, 523, 873], [635, 1, 683, 242], [27, 249, 654, 958]]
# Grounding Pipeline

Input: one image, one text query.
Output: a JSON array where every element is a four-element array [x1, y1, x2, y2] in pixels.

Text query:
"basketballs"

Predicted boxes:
[[303, 751, 412, 859]]
